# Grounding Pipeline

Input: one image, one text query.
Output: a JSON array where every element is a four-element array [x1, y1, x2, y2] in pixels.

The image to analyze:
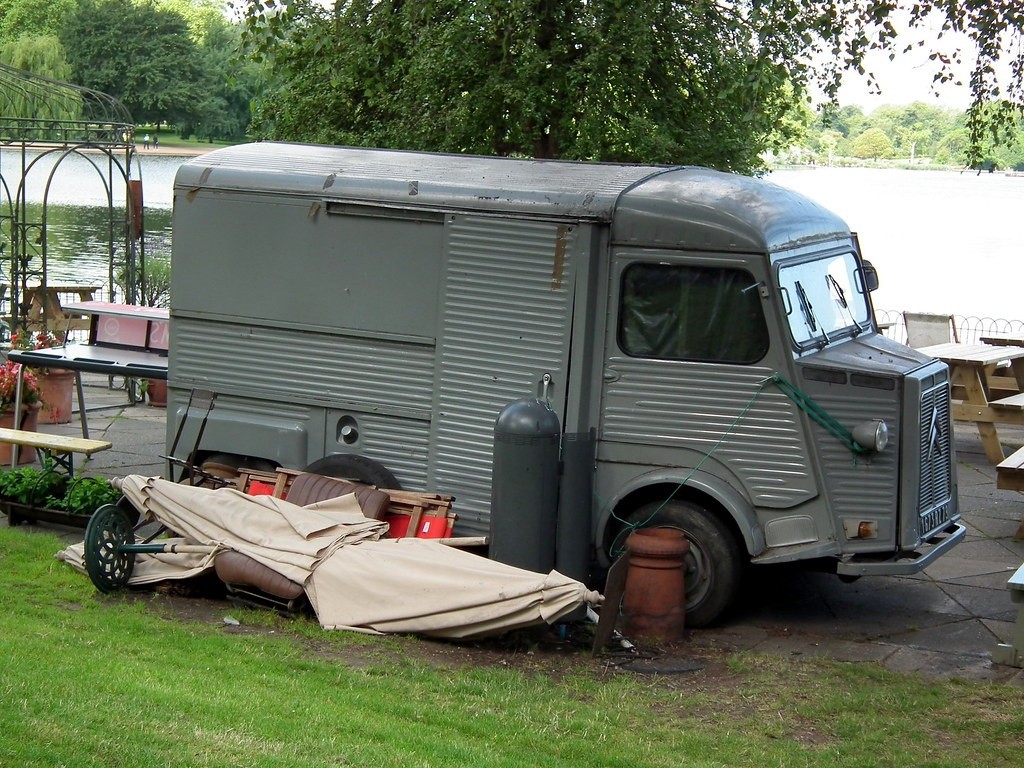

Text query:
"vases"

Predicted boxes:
[[0, 400, 44, 465], [35, 369, 76, 424]]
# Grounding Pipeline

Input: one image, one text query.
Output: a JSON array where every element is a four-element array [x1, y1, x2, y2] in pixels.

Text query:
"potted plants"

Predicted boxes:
[[129, 376, 167, 408]]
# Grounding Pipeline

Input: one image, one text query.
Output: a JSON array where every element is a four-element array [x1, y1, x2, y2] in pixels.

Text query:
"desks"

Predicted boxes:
[[981, 334, 1024, 393], [914, 341, 1024, 468], [879, 322, 896, 336], [10, 300, 170, 469], [0, 281, 104, 346]]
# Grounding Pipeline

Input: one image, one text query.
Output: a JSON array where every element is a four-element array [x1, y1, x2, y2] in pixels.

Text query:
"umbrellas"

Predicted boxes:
[[55, 473, 604, 639]]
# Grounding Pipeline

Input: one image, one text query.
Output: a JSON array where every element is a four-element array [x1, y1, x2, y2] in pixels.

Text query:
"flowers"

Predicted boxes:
[[0, 360, 45, 413], [7, 327, 66, 377]]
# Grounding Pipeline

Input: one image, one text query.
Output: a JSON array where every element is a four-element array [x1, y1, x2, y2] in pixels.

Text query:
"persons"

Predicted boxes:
[[144, 134, 150, 150], [122, 129, 132, 149], [153, 134, 159, 151]]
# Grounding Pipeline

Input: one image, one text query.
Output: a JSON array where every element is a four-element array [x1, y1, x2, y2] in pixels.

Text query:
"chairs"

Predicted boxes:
[[214, 471, 392, 621], [158, 386, 237, 489], [235, 469, 459, 541], [902, 312, 961, 348], [678, 273, 757, 361]]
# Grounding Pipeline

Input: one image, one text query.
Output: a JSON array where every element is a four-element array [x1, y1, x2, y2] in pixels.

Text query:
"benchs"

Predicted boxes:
[[0, 427, 112, 480], [989, 391, 1024, 411], [996, 444, 1024, 492]]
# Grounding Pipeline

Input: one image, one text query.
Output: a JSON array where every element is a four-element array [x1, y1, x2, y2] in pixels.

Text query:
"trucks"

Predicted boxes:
[[162, 141, 970, 634]]
